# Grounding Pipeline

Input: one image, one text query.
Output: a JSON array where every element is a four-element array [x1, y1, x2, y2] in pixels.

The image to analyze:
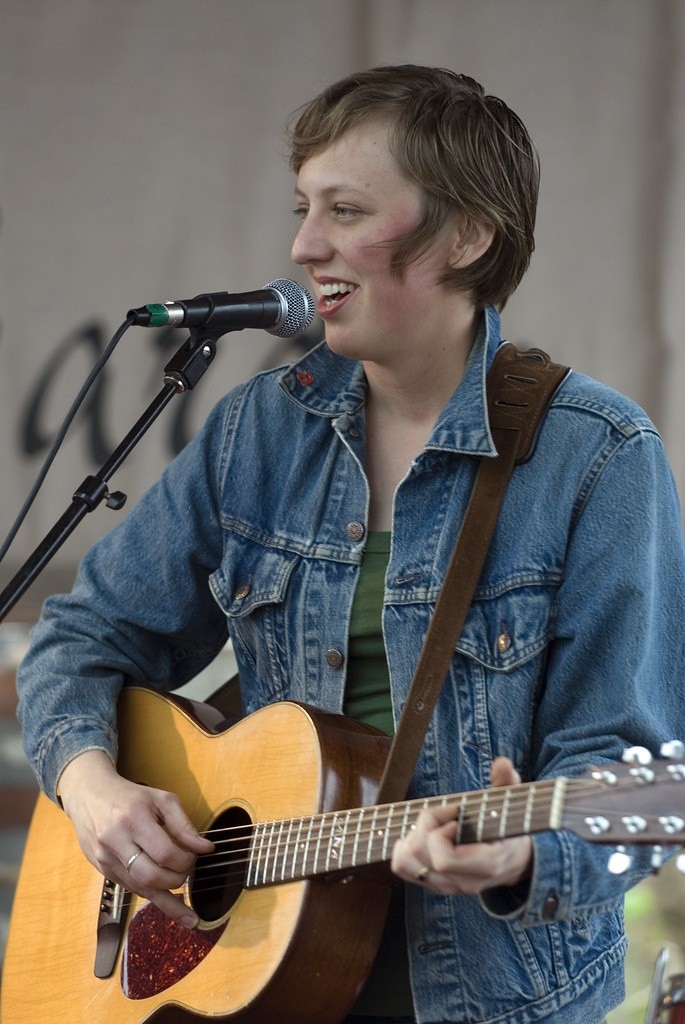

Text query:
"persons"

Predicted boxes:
[[14, 64, 685, 1024]]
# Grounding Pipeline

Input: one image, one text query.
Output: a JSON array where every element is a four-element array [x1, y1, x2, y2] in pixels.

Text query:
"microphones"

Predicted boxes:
[[128, 276, 315, 337]]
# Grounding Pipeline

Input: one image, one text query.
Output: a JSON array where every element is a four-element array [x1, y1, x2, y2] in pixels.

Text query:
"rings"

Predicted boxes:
[[411, 867, 433, 886], [124, 849, 142, 869]]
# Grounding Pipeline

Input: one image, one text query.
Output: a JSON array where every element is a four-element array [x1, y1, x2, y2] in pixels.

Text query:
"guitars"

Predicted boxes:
[[1, 685, 685, 1024]]
